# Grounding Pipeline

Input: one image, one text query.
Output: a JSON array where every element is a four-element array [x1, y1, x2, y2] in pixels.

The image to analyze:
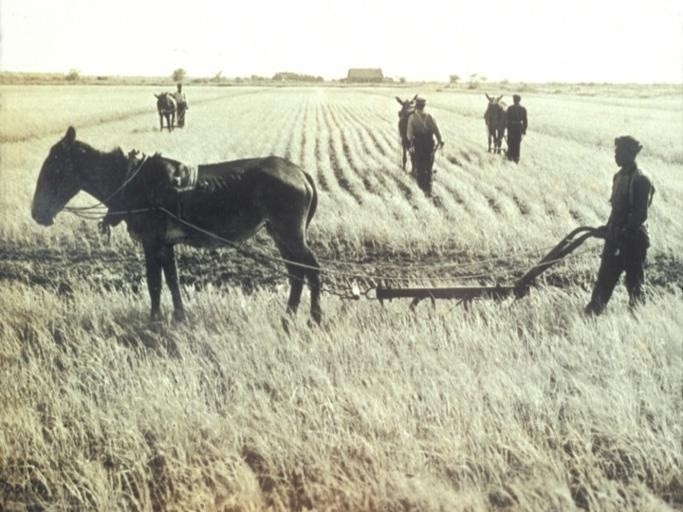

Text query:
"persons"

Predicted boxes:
[[500, 92, 528, 164], [168, 81, 190, 129], [582, 131, 654, 315], [404, 97, 443, 199]]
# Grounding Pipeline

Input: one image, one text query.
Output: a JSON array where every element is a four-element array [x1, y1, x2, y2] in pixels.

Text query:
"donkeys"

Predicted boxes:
[[395, 94, 417, 173], [154, 91, 179, 133], [31, 126, 323, 332], [484, 93, 508, 154]]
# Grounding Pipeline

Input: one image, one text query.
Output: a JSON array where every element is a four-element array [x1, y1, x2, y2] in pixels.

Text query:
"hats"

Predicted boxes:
[[415, 97, 426, 106], [612, 135, 643, 153]]
